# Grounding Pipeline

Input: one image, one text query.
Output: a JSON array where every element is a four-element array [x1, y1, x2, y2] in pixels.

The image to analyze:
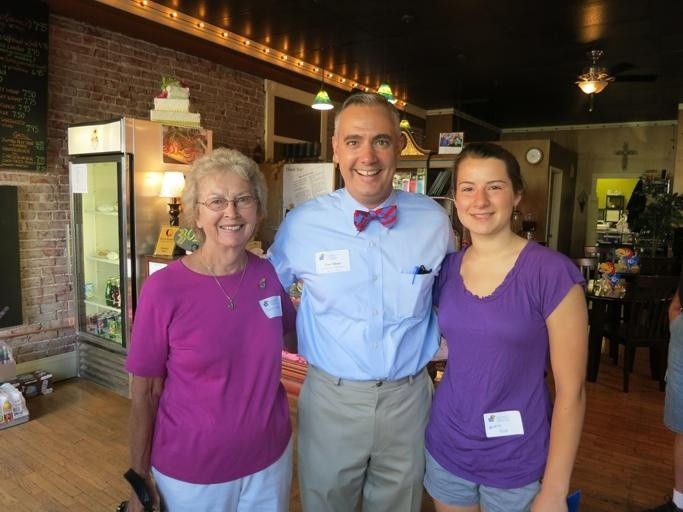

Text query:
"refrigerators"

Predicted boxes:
[[65, 118, 213, 401]]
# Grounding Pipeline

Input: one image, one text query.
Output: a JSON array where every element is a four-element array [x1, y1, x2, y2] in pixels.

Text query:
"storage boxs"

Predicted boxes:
[[149, 85, 200, 127], [17, 368, 54, 400]]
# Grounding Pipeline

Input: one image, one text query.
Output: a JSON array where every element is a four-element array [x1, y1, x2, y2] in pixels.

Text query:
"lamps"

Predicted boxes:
[[158, 171, 186, 257], [306, 46, 415, 136], [575, 50, 614, 113]]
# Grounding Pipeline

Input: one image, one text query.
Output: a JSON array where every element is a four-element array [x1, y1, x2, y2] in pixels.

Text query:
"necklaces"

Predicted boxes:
[[194, 245, 249, 310]]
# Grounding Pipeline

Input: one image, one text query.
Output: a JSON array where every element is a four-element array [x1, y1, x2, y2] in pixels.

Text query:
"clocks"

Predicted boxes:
[[525, 147, 544, 165]]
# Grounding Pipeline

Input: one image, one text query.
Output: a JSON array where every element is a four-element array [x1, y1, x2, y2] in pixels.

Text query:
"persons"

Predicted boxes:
[[429, 141, 589, 512], [644, 282, 683, 512], [263, 92, 457, 512], [126, 147, 300, 512]]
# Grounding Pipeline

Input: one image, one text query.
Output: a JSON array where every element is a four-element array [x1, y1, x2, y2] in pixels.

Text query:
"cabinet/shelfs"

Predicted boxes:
[[596, 195, 627, 228]]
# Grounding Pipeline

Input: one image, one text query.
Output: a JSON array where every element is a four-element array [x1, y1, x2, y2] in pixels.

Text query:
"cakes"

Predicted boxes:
[[150, 77, 201, 127]]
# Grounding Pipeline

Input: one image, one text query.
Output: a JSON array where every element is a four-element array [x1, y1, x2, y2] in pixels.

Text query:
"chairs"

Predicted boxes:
[[564, 230, 683, 393]]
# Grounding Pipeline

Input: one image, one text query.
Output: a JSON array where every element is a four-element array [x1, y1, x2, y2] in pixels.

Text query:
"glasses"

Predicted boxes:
[[196, 195, 259, 211]]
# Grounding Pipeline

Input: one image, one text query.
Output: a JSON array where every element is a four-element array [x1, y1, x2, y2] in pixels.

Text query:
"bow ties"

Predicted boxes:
[[353, 206, 397, 231]]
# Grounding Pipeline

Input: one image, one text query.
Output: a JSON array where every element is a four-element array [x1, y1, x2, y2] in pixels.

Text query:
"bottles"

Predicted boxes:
[[510, 210, 538, 241], [105, 277, 123, 308]]
